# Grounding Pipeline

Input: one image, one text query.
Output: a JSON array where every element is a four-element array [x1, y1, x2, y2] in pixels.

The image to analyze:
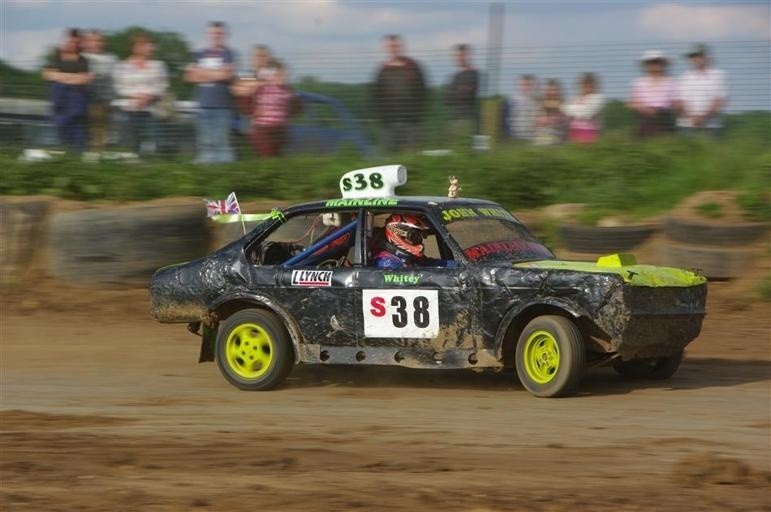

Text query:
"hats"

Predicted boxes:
[[684, 43, 708, 56], [639, 46, 674, 65]]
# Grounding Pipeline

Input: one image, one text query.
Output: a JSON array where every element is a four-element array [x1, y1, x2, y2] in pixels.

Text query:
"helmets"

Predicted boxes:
[[383, 214, 426, 258]]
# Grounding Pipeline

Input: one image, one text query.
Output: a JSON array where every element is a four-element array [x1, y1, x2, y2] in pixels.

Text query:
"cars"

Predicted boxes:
[[108, 87, 371, 161], [148, 164, 709, 396]]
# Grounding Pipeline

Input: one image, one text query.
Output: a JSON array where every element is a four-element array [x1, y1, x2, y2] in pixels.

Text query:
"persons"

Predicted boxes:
[[373, 212, 447, 271]]
